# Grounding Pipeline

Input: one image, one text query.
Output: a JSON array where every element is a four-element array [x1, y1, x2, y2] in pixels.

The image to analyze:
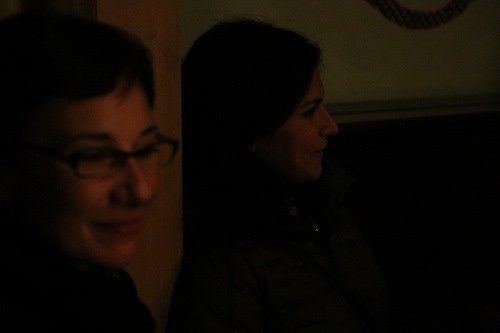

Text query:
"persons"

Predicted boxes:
[[0, 13, 178, 333], [165, 19, 390, 333]]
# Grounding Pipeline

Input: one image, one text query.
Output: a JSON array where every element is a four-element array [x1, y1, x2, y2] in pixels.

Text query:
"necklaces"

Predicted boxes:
[[243, 186, 320, 233]]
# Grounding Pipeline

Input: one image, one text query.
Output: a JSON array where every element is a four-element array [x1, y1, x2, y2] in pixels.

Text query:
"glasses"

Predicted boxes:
[[24, 132, 178, 181]]
